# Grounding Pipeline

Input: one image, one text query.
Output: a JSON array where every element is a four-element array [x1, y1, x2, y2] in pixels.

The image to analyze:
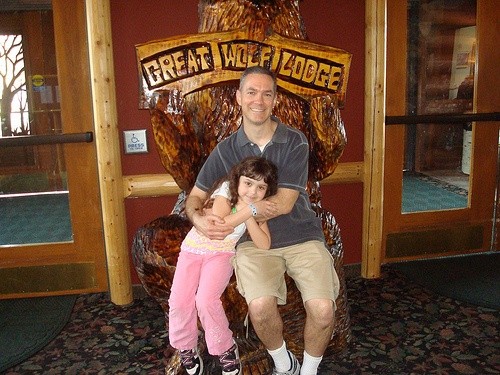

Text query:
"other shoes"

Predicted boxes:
[[272, 350, 300, 375]]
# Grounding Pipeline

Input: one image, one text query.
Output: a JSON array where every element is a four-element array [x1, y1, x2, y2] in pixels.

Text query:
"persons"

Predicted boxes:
[[186, 65, 340, 375], [169, 157, 279, 375]]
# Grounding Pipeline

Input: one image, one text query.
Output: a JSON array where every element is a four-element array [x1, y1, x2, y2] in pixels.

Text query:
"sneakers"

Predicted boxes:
[[217, 339, 242, 375], [175, 345, 204, 375]]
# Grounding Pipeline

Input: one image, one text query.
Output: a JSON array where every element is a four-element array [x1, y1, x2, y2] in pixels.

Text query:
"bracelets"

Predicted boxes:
[[246, 206, 257, 217], [232, 207, 236, 214]]
